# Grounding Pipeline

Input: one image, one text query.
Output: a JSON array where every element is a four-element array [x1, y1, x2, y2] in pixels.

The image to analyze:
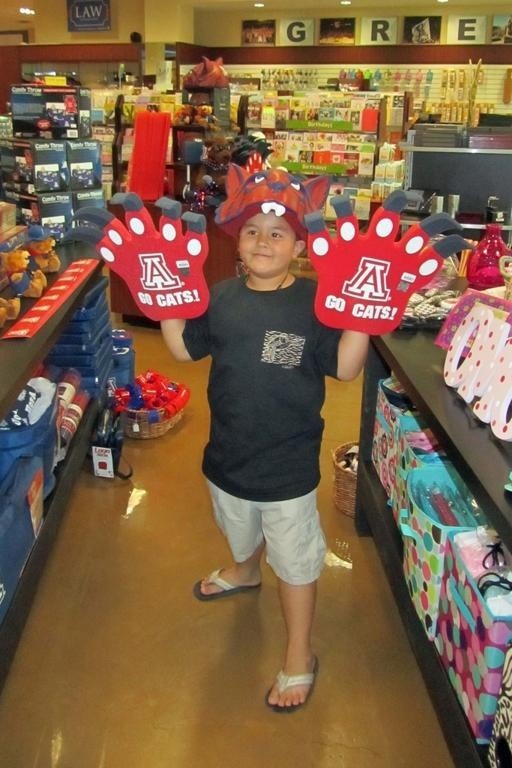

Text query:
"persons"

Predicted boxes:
[[65, 160, 475, 712]]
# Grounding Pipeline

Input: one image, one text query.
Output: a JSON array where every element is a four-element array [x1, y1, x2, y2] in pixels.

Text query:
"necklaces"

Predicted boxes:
[[275, 273, 290, 291]]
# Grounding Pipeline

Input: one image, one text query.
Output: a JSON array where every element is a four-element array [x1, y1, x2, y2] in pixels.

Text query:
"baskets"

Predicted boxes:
[[124, 380, 185, 440], [330, 441, 359, 518]]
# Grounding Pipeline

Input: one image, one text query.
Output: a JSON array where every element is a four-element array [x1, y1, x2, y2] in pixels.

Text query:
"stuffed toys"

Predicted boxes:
[[0, 224, 60, 332]]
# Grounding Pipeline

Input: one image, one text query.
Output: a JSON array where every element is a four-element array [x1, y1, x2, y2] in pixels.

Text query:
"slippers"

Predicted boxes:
[[266, 653, 318, 712], [193, 568, 262, 601]]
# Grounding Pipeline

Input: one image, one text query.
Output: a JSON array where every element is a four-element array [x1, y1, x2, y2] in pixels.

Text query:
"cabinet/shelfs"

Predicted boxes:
[[0, 240, 102, 696], [106, 92, 240, 328], [353, 327, 512, 766], [234, 89, 414, 229]]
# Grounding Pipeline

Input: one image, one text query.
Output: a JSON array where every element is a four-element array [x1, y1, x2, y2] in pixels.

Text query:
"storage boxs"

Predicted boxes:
[[370, 376, 450, 528], [0, 83, 105, 241], [433, 527, 512, 745], [402, 464, 489, 642], [486, 638, 512, 768]]
